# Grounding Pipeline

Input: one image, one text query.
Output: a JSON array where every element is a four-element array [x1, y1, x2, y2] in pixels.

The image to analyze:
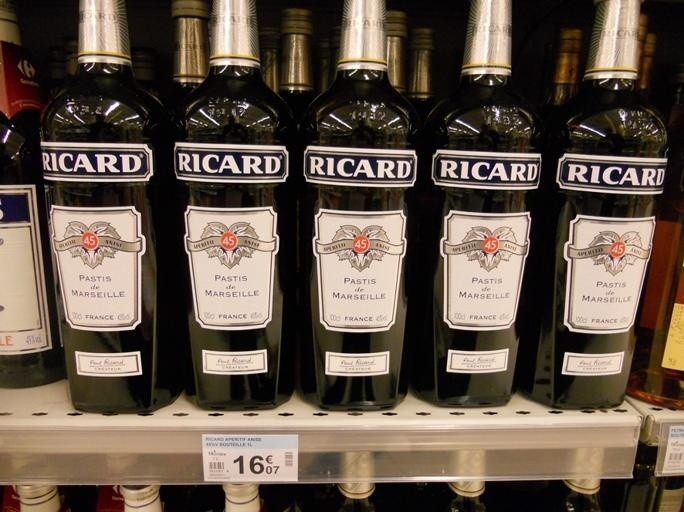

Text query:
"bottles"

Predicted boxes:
[[383, 8, 410, 95], [171, 0, 297, 412], [308, 13, 344, 97], [634, 13, 658, 104], [418, 1, 545, 409], [39, 0, 191, 414], [0, 1, 68, 390], [258, 8, 303, 95], [414, 22, 439, 108], [4, 448, 684, 512], [625, 106, 684, 410], [174, 1, 211, 114], [518, 1, 671, 409], [132, 45, 157, 97], [547, 27, 587, 111], [304, 0, 419, 411]]
